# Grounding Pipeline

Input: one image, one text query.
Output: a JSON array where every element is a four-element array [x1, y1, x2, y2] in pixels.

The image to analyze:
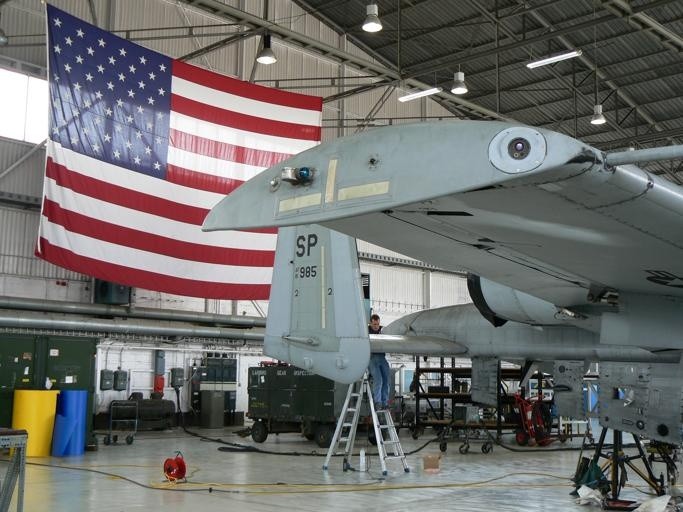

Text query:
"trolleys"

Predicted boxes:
[[92, 400, 137, 445], [511, 390, 573, 448], [438, 418, 493, 454]]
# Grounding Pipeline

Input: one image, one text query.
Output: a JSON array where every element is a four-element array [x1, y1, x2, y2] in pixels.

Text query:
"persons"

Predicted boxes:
[[367, 313, 393, 410]]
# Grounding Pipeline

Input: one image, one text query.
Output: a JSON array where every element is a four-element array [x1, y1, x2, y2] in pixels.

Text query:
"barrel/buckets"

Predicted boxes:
[[421, 453, 442, 474]]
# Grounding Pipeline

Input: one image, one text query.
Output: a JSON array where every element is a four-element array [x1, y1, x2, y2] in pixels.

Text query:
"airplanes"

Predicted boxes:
[[199, 121, 682, 485]]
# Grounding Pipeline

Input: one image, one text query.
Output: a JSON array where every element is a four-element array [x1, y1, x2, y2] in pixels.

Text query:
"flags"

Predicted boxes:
[[32, 1, 326, 303]]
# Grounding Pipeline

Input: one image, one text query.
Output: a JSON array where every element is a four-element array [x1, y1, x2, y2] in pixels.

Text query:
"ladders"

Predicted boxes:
[[322, 373, 410, 475]]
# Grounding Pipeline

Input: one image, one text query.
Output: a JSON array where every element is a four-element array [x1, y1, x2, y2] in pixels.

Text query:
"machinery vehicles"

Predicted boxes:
[[238, 358, 399, 448]]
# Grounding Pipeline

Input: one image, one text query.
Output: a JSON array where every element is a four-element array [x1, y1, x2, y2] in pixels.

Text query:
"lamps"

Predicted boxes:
[[362, 3, 382, 33], [398, 72, 443, 103], [450, 64, 469, 95], [590, 6, 607, 125], [255, 34, 277, 65], [525, 24, 582, 69]]
